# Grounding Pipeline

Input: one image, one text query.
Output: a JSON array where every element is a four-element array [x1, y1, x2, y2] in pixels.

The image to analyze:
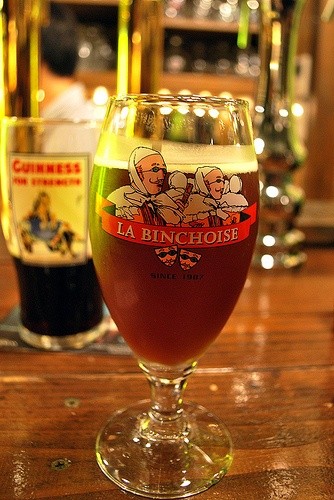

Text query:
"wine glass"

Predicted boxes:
[[86, 94, 260, 500]]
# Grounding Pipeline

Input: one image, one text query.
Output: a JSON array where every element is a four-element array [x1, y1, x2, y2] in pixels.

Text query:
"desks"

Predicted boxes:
[[0, 247, 334, 500]]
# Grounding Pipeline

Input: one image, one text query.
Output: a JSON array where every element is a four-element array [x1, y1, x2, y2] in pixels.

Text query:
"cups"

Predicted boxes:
[[0, 117, 108, 351]]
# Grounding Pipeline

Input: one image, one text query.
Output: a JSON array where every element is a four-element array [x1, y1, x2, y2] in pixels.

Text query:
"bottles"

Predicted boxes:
[[253, 16, 307, 270]]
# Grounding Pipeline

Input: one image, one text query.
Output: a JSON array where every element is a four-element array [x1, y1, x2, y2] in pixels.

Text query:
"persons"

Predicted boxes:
[[25, 25, 100, 158]]
